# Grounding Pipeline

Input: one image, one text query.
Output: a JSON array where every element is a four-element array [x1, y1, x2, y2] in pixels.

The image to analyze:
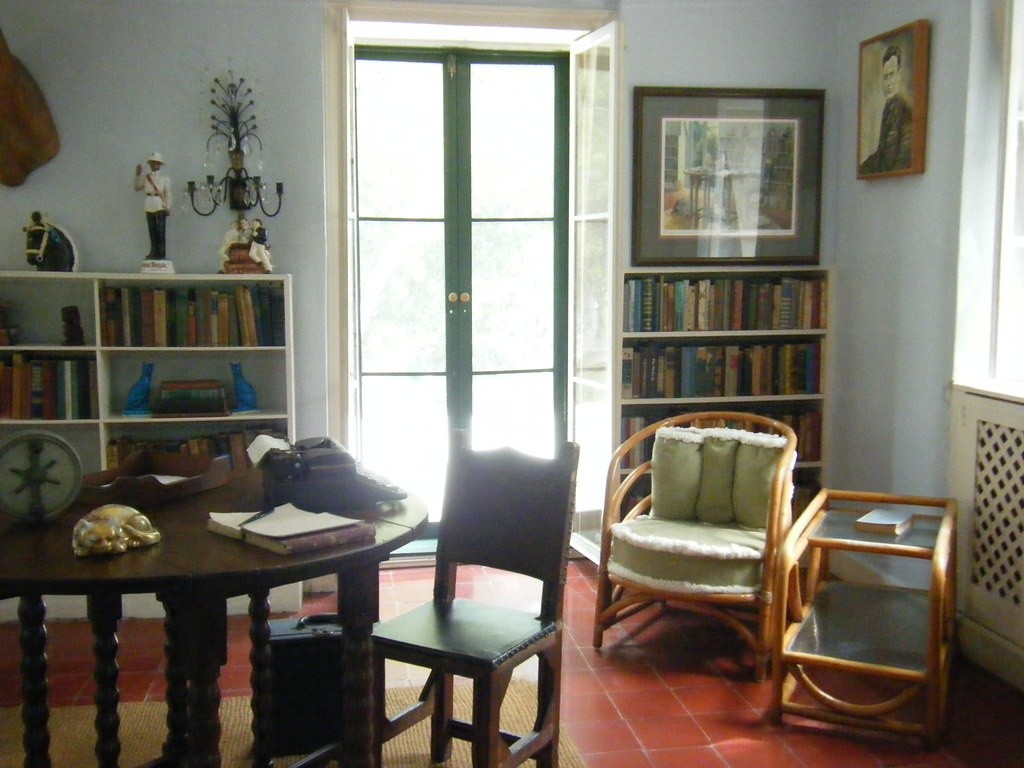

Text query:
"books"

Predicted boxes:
[[856, 505, 915, 535], [0, 278, 376, 556], [621, 274, 823, 470]]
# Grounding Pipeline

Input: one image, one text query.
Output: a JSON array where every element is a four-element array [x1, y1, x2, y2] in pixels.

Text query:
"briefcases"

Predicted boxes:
[[268, 611, 386, 754]]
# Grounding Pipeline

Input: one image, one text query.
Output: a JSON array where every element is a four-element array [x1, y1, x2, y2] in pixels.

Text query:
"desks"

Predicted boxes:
[[0, 468, 429, 768]]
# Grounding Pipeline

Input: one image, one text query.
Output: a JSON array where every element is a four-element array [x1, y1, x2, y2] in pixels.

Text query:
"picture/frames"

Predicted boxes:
[[630, 86, 824, 266], [857, 19, 931, 180]]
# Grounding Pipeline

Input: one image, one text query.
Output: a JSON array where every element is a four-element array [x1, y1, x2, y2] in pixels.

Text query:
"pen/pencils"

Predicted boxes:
[[238, 505, 275, 527]]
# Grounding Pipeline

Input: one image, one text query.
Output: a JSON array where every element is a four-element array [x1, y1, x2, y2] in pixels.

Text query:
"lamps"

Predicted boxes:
[[184, 68, 286, 273]]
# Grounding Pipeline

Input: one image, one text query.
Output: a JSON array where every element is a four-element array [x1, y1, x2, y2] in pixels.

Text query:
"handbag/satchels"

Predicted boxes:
[[256, 447, 356, 513]]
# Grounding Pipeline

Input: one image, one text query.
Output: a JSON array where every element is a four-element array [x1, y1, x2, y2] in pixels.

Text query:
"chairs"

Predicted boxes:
[[593, 410, 798, 683], [371, 429, 579, 768]]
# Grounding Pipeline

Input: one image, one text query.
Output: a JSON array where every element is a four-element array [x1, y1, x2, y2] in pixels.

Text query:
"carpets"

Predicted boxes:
[[0, 678, 589, 768]]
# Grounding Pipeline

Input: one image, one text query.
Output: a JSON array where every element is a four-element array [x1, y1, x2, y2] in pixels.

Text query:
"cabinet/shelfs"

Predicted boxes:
[[617, 271, 829, 526], [772, 487, 960, 747], [0, 270, 303, 621]]
